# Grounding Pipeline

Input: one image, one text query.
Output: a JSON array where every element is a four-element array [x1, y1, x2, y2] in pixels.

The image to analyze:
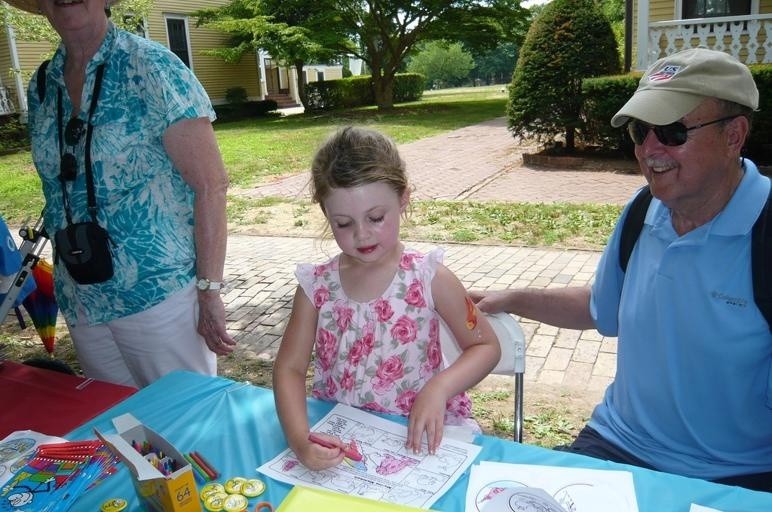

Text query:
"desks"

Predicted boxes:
[[1, 355, 139, 441], [41, 366, 771, 512]]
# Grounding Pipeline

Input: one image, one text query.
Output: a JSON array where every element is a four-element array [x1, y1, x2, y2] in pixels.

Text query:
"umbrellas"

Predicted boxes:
[[17, 225, 60, 360]]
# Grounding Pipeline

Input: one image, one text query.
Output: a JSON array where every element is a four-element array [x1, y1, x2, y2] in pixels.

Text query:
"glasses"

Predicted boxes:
[[628, 115, 745, 144]]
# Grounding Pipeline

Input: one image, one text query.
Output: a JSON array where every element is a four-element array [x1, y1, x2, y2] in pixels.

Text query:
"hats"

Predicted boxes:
[[610, 49, 760, 128]]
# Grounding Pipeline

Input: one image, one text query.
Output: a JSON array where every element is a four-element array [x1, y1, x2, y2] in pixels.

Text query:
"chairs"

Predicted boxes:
[[438, 299, 526, 443]]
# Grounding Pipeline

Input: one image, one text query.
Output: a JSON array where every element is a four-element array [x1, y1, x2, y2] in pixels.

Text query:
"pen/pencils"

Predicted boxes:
[[132, 439, 182, 477], [308, 435, 362, 461], [183, 451, 221, 482]]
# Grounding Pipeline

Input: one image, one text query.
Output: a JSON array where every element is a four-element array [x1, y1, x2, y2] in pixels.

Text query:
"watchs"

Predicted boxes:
[[195, 276, 224, 291]]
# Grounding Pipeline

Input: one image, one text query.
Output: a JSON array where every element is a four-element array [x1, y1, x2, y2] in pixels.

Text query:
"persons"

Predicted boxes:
[[6, 2, 236, 392], [467, 50, 771, 491], [271, 124, 502, 468]]
